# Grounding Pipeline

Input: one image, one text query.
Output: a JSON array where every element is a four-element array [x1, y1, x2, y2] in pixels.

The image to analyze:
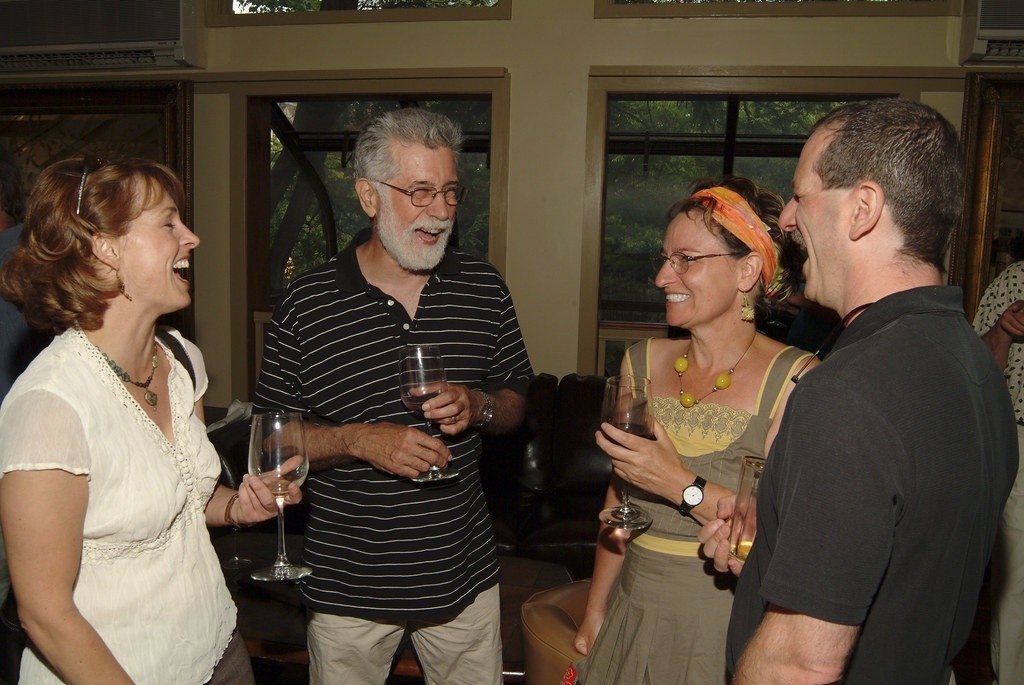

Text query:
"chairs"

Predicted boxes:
[[476, 370, 615, 545]]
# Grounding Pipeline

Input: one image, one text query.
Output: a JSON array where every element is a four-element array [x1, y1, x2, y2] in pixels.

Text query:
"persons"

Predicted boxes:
[[697, 93, 1019, 685], [252, 108, 539, 684], [0, 154, 305, 685], [574, 178, 822, 684], [973, 259, 1024, 685]]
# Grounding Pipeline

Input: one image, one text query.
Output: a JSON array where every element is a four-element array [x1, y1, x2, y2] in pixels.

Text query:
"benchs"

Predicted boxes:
[[211, 534, 574, 685]]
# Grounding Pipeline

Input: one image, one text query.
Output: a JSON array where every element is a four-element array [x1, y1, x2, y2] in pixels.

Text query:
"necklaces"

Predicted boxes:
[[675, 330, 757, 407], [93, 341, 159, 412]]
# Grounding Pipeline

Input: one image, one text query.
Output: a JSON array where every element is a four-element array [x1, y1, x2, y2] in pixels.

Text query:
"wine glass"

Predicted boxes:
[[398, 348, 459, 482], [247, 412, 313, 582], [598, 376, 656, 530]]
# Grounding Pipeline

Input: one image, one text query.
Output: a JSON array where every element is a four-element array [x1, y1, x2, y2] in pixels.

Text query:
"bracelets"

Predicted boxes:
[[224, 493, 257, 530]]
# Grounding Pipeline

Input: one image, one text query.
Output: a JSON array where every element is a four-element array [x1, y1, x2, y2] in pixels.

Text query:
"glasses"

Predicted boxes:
[[374, 178, 469, 208], [75, 154, 107, 217], [656, 252, 745, 275]]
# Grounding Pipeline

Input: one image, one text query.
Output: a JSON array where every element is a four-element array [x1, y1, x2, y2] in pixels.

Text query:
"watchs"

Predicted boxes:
[[474, 391, 494, 428], [678, 475, 708, 516]]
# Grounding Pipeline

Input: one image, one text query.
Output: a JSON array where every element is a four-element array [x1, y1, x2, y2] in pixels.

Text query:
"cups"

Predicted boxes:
[[724, 457, 768, 565]]
